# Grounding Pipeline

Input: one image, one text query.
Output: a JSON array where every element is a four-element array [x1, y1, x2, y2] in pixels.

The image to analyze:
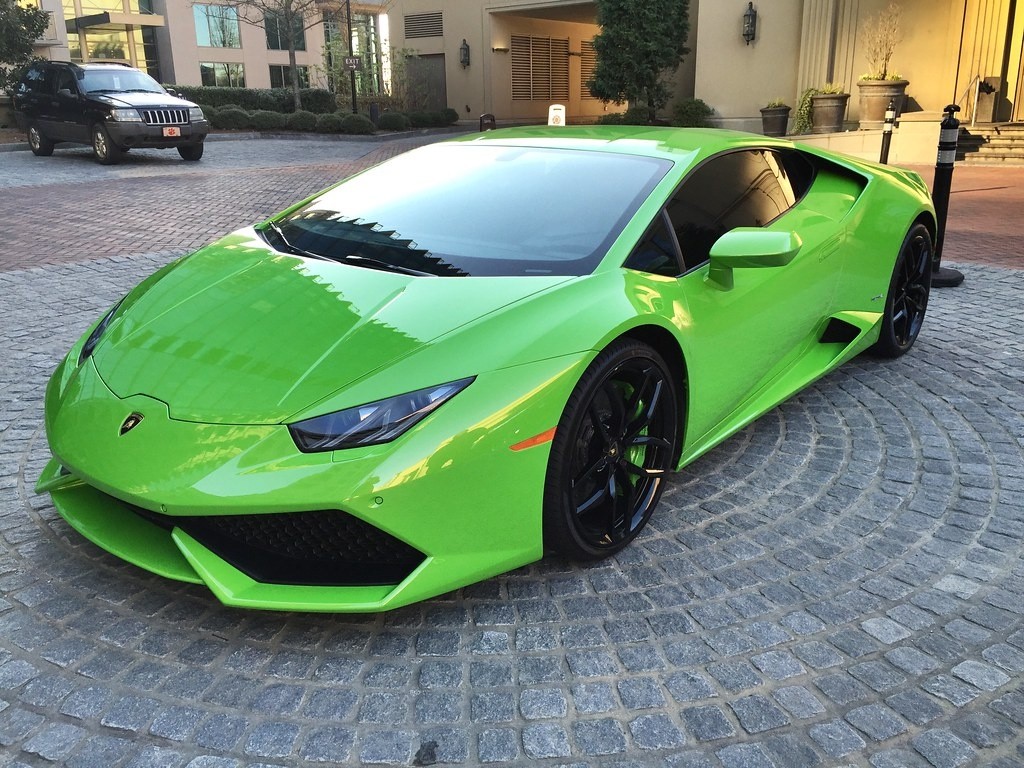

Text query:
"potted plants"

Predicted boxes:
[[787, 82, 852, 136], [977, 81, 998, 123], [760, 98, 792, 137], [856, 2, 910, 129]]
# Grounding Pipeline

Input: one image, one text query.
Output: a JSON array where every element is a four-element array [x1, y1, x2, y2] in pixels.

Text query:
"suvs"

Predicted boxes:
[[13, 59, 210, 165]]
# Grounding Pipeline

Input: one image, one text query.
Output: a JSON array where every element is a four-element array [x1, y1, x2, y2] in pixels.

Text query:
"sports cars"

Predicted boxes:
[[35, 126, 938, 612]]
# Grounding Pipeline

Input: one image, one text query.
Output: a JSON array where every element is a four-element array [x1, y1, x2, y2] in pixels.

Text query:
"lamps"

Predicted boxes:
[[460, 38, 470, 69], [743, 2, 757, 45], [491, 47, 509, 53]]
